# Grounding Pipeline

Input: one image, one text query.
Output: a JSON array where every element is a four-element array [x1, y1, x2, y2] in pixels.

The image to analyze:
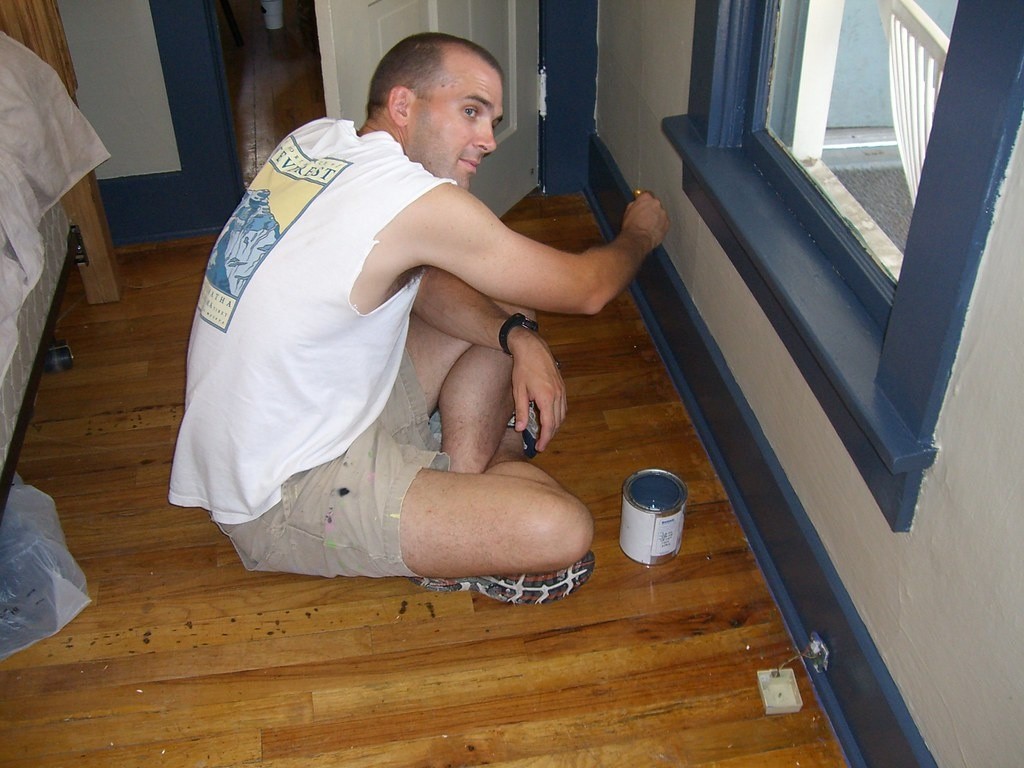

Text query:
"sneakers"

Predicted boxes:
[[407, 547, 597, 605], [508, 398, 545, 459]]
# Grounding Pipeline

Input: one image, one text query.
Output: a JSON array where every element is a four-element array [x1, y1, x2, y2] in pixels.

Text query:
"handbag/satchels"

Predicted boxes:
[[0, 472, 93, 663]]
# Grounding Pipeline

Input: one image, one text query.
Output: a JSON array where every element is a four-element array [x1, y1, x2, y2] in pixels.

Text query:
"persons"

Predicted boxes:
[[167, 32, 671, 604]]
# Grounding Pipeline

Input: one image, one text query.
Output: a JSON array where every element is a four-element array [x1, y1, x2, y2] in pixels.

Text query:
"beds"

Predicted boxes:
[[0, 0, 123, 526]]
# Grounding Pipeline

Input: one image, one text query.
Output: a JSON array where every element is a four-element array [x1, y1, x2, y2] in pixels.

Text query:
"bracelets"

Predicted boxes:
[[500, 313, 539, 356]]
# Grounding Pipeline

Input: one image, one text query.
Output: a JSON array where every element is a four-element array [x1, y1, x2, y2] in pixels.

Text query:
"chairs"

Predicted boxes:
[[878, 0, 951, 210]]
